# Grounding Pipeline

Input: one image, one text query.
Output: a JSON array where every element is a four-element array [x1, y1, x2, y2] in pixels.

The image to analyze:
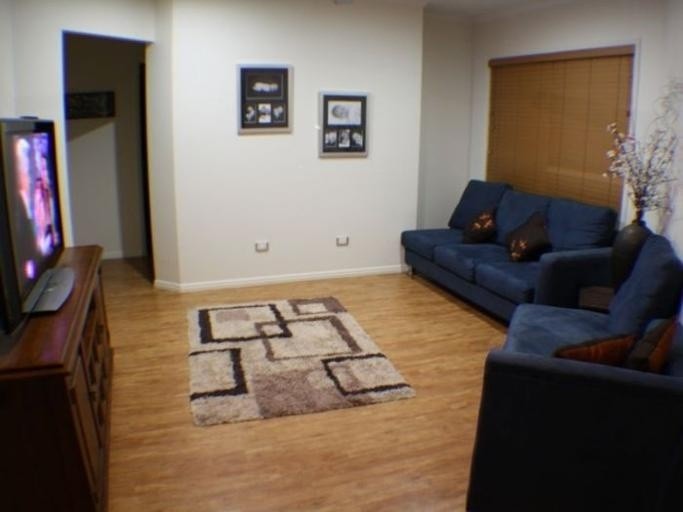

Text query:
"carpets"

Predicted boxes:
[[185, 296, 416, 426]]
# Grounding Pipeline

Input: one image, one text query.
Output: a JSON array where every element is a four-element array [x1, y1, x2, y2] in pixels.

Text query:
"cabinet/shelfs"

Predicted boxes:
[[0, 244, 114, 512]]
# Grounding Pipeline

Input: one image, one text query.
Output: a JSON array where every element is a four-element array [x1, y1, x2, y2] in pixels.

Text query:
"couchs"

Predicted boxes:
[[401, 180, 617, 324], [464, 235, 683, 512]]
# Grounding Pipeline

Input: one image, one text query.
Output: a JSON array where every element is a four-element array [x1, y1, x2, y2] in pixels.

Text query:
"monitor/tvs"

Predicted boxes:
[[0, 118, 74, 346]]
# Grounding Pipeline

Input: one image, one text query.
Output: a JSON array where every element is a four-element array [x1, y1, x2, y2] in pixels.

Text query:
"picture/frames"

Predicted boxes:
[[234, 63, 295, 135], [317, 90, 370, 158]]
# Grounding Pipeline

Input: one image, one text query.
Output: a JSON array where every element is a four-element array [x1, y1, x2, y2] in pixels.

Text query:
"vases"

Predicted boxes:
[[611, 220, 653, 295]]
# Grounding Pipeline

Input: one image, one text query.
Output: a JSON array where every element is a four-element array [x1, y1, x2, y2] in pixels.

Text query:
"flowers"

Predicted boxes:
[[601, 79, 683, 220]]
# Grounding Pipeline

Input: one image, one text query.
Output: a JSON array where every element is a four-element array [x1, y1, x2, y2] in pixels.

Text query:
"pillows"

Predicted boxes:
[[505, 209, 553, 263], [550, 331, 636, 366], [622, 318, 679, 374], [462, 203, 498, 245]]
